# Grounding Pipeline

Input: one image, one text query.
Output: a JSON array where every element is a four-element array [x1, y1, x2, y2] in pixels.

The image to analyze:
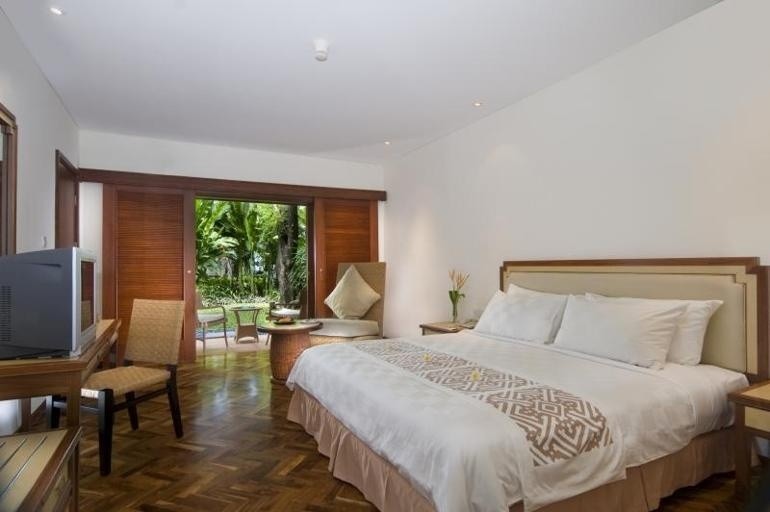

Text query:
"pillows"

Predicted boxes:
[[471, 289, 562, 345], [584, 290, 725, 366], [552, 295, 691, 370], [507, 282, 584, 343], [323, 262, 382, 319]]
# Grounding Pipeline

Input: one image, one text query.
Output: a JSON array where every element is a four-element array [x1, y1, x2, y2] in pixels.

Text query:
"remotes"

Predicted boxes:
[[274, 321, 295, 325]]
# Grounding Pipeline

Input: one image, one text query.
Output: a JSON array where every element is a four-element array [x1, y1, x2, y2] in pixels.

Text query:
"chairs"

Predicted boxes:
[[265, 285, 307, 347], [196, 289, 230, 350], [44, 296, 188, 478], [303, 260, 387, 346]]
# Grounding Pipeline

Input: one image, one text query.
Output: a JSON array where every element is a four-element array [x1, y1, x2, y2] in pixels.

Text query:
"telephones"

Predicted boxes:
[[459, 318, 478, 329]]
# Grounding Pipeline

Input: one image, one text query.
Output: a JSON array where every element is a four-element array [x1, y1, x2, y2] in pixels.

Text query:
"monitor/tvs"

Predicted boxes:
[[0, 247, 98, 361]]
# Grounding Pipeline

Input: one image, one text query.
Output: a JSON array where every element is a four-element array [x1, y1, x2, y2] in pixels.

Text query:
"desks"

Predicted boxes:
[[0, 317, 123, 429], [256, 319, 323, 386], [230, 305, 263, 344]]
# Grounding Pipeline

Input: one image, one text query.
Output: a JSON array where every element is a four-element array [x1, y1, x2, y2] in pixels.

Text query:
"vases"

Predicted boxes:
[[447, 289, 466, 325]]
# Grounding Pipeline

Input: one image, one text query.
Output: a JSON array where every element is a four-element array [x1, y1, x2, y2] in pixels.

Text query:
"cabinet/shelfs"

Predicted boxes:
[[1, 425, 83, 511]]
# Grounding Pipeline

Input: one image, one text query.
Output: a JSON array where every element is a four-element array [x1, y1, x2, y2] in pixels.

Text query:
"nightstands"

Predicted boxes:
[[419, 319, 476, 337], [727, 378, 770, 501]]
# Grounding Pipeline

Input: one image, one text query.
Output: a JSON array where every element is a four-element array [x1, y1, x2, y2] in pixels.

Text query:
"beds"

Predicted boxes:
[[285, 255, 770, 512]]
